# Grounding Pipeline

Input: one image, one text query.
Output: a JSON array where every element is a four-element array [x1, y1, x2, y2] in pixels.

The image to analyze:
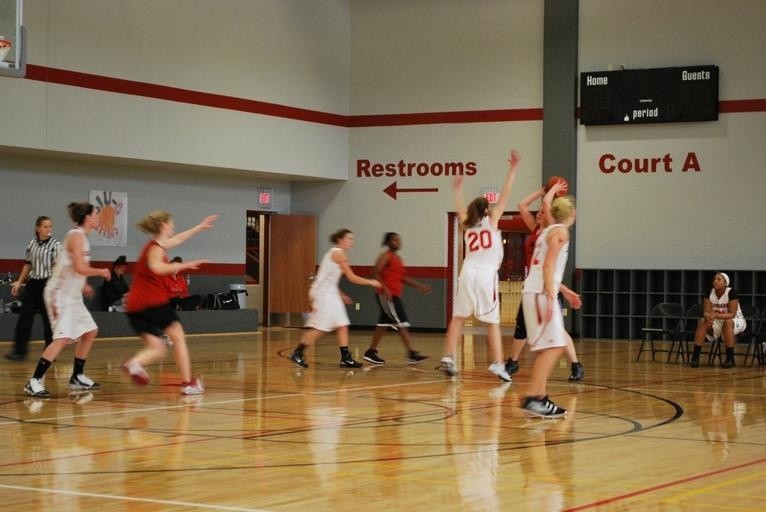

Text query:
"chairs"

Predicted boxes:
[[635, 300, 766, 367]]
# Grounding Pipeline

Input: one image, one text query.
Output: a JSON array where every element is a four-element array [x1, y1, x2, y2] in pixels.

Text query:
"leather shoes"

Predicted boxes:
[[3, 353, 29, 362]]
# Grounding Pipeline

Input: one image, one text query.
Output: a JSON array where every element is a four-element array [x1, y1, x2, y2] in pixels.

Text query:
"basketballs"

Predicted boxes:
[[544, 177, 568, 197]]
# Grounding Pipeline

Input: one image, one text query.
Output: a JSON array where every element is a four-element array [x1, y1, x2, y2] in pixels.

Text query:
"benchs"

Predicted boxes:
[[0, 270, 259, 343]]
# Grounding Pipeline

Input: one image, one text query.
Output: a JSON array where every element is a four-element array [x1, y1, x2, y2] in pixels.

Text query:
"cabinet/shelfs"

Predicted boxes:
[[578, 268, 766, 346]]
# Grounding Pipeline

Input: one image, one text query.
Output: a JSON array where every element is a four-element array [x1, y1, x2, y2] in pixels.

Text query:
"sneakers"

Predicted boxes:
[[723, 359, 736, 368], [361, 351, 384, 364], [119, 357, 151, 385], [339, 359, 363, 368], [691, 356, 699, 367], [441, 356, 460, 376], [24, 380, 50, 397], [406, 351, 429, 363], [568, 362, 584, 380], [182, 379, 205, 394], [66, 373, 100, 389], [545, 397, 567, 417], [487, 361, 513, 381], [290, 349, 311, 368], [505, 358, 519, 372], [520, 398, 554, 418]]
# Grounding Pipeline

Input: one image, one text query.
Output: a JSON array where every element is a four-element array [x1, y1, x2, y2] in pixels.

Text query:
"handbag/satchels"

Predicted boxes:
[[202, 292, 240, 310]]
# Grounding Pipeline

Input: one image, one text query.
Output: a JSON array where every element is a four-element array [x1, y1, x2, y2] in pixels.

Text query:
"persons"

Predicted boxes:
[[291, 229, 382, 369], [504, 181, 584, 382], [165, 256, 202, 311], [20, 200, 103, 395], [362, 232, 434, 368], [365, 371, 424, 499], [446, 375, 577, 512], [101, 252, 131, 314], [9, 361, 189, 512], [440, 149, 522, 384], [518, 196, 581, 417], [686, 270, 748, 370], [296, 368, 349, 496], [120, 208, 218, 396], [3, 217, 61, 360], [690, 378, 747, 466]]
